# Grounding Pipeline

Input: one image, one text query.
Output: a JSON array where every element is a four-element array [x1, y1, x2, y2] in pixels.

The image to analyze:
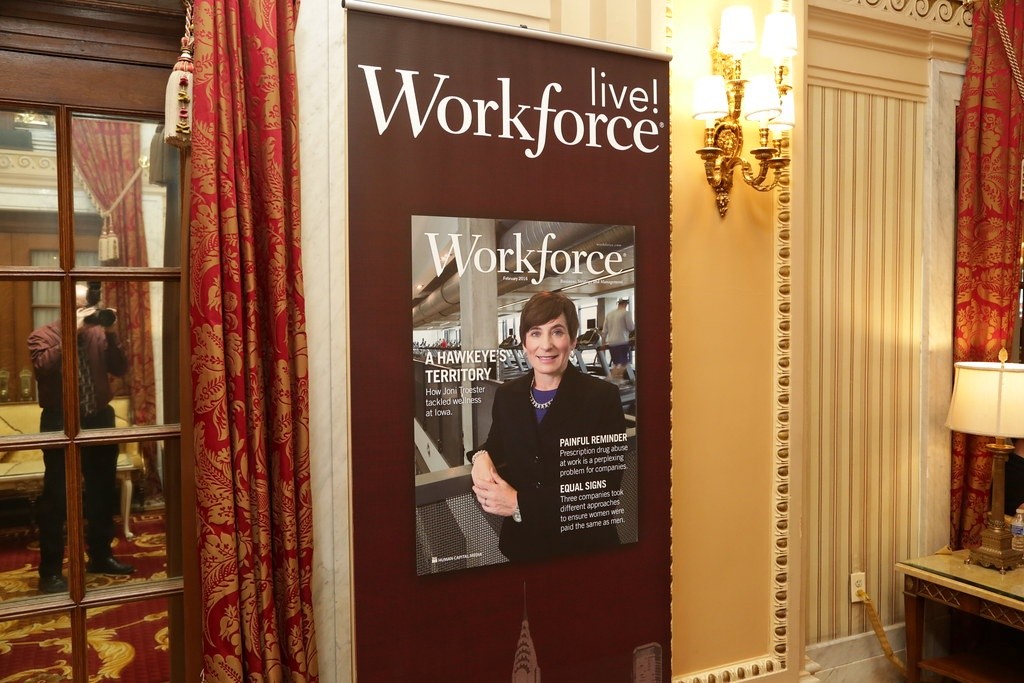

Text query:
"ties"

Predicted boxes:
[[77, 333, 98, 417]]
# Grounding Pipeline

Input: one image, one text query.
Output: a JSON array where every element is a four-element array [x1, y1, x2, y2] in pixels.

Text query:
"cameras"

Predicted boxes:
[[82, 281, 116, 327]]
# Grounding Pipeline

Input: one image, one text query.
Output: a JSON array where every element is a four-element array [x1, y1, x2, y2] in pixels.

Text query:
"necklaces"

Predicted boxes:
[[529, 376, 553, 409]]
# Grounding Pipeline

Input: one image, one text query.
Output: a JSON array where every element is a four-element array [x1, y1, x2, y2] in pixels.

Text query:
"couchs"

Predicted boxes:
[[0, 396, 146, 500]]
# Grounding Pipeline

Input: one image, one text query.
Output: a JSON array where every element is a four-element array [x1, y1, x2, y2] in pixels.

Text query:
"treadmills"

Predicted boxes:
[[413, 330, 636, 407]]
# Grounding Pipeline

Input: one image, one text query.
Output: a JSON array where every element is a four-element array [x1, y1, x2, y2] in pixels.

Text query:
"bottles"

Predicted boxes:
[[1010, 508, 1024, 551]]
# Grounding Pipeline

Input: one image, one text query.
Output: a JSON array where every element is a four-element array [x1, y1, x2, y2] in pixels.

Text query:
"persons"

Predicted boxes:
[[27, 284, 137, 592], [465, 292, 626, 560], [600, 302, 635, 378], [438, 339, 446, 348]]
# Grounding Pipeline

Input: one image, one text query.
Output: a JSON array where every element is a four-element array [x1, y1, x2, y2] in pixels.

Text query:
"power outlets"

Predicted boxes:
[[851, 572, 866, 603]]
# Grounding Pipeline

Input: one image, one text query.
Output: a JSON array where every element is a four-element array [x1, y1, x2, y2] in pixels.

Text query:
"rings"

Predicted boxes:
[[484, 498, 487, 505]]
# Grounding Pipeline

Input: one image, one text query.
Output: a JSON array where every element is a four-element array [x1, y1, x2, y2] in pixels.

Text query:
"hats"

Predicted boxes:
[[618, 296, 630, 304]]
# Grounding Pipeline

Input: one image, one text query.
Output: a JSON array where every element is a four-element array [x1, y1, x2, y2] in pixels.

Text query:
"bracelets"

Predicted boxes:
[[472, 449, 488, 464]]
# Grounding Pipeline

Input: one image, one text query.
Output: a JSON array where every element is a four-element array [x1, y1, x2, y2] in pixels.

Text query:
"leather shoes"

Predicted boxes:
[[85, 554, 133, 575], [39, 574, 68, 593]]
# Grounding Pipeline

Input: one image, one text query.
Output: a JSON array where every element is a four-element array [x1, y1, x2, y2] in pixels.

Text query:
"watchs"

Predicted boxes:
[[512, 503, 522, 522]]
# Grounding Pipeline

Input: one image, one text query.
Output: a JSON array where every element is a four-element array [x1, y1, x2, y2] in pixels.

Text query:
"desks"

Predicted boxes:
[[894, 549, 1024, 683], [0, 453, 145, 539]]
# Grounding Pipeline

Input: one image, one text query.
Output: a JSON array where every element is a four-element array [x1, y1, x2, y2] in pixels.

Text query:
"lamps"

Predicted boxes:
[[945, 347, 1024, 574], [690, 1, 795, 220]]
